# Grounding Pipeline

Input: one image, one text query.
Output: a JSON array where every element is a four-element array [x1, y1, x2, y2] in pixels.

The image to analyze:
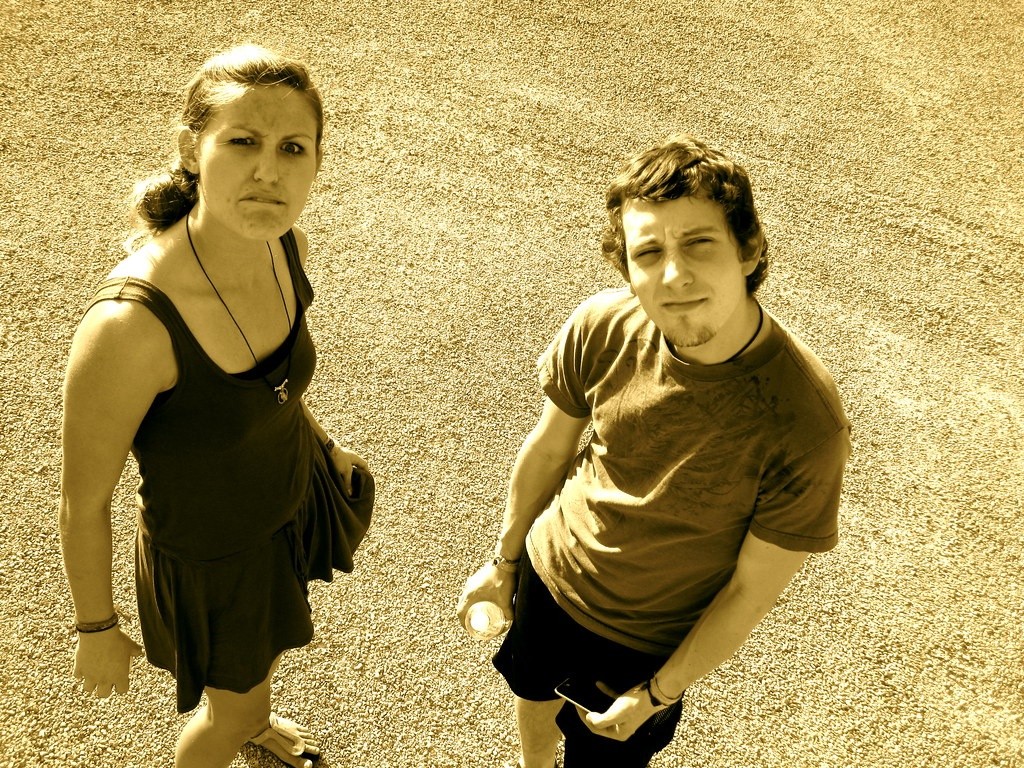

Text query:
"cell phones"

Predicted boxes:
[[554, 677, 615, 716]]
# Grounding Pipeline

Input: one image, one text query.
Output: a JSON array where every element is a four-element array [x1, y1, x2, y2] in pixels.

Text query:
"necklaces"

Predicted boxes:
[[186, 212, 297, 405], [719, 298, 766, 365]]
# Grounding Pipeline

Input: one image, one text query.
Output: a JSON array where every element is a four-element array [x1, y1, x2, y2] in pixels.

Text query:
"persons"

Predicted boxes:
[[457, 136, 852, 768], [57, 42, 378, 768]]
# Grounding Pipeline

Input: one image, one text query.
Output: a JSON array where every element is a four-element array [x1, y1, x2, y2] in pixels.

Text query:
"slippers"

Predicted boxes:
[[249, 712, 321, 768]]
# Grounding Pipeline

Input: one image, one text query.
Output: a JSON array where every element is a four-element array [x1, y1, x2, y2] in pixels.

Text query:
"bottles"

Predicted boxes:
[[465, 601, 511, 639]]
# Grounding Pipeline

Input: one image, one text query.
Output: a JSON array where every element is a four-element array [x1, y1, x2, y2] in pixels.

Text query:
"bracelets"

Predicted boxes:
[[490, 553, 520, 573], [322, 435, 338, 455], [638, 676, 683, 709], [75, 612, 120, 632]]
